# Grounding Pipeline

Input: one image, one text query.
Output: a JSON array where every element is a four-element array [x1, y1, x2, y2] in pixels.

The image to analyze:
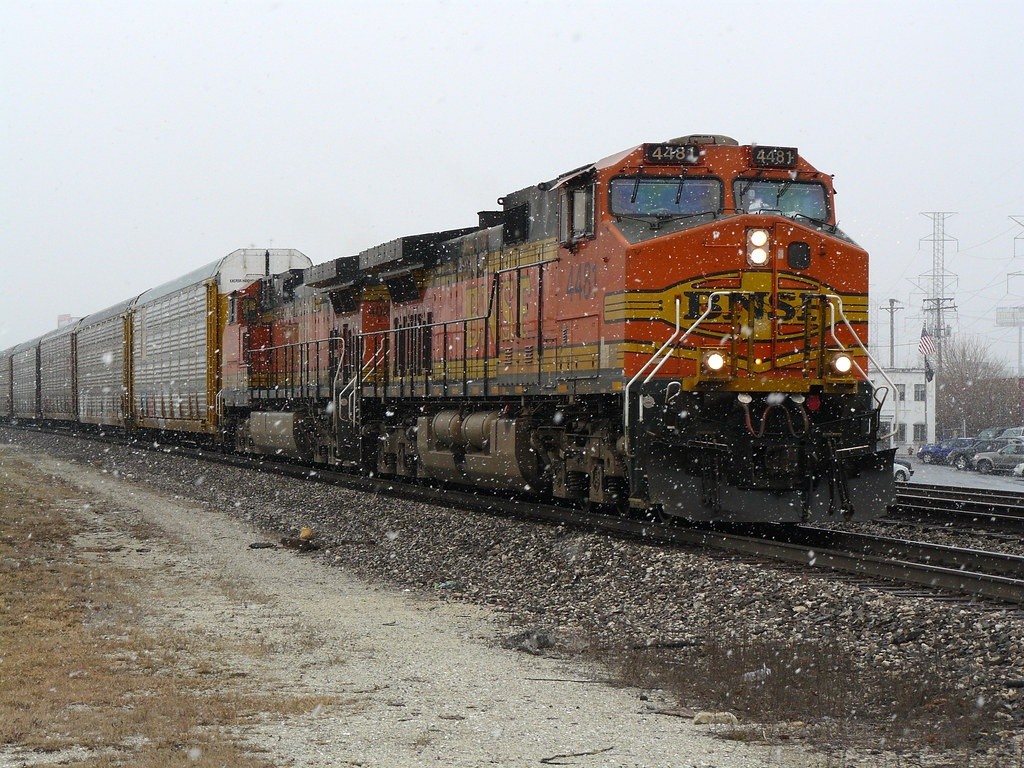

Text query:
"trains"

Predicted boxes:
[[0, 133, 900, 526]]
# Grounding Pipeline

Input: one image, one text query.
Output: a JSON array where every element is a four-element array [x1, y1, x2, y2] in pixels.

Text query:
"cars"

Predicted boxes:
[[1013, 463, 1024, 479], [894, 459, 914, 477], [917, 438, 975, 464], [971, 444, 1024, 474], [893, 462, 911, 483]]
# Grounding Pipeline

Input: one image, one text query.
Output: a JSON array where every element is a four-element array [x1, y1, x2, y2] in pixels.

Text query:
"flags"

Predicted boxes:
[[925, 361, 934, 383], [918, 329, 937, 355]]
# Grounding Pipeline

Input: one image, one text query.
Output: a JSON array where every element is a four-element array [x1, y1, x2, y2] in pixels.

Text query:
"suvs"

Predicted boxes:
[[945, 438, 1024, 471]]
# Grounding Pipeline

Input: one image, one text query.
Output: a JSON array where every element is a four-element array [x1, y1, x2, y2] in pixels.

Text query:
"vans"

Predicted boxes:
[[975, 427, 1024, 438]]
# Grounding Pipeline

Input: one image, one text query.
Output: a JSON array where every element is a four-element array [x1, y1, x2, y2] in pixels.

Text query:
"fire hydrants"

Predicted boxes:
[[907, 447, 914, 455]]
